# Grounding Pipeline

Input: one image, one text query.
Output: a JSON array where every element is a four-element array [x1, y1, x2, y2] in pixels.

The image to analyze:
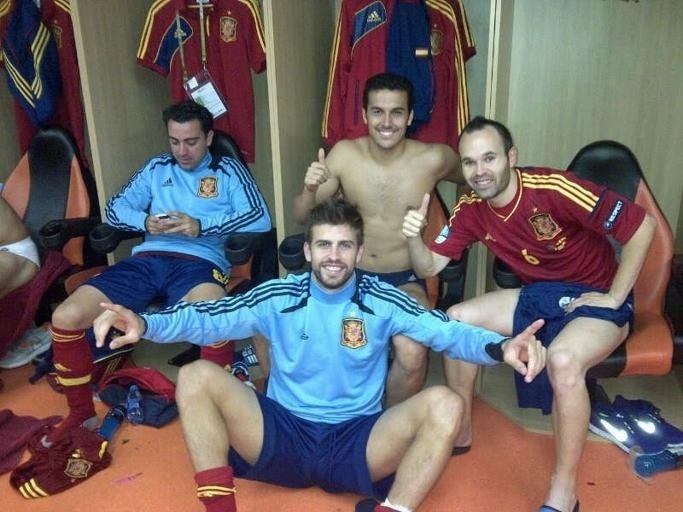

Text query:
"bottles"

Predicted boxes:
[[97, 385, 142, 442], [634, 450, 681, 478]]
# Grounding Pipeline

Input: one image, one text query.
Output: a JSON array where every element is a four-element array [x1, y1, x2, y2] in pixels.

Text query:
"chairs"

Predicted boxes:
[[0, 121, 103, 327], [488, 137, 683, 424], [277, 188, 466, 316], [88, 126, 257, 364]]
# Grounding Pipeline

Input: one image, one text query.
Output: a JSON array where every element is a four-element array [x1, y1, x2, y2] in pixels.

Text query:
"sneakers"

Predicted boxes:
[[0, 322, 52, 369], [587, 394, 683, 458]]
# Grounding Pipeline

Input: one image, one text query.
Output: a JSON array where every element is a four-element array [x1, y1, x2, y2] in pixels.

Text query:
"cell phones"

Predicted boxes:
[[155, 214, 170, 223]]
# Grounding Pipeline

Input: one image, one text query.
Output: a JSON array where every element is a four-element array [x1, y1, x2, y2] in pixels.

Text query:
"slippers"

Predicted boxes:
[[538, 499, 579, 512]]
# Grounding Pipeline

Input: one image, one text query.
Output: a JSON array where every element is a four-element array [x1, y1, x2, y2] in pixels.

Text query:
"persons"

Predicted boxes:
[[31, 100, 272, 458], [0, 192, 42, 300], [294, 72, 467, 411], [93, 196, 547, 511], [401, 116, 658, 511]]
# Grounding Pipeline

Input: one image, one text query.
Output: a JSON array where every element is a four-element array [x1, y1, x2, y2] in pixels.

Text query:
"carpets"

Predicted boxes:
[[1, 363, 683, 512]]
